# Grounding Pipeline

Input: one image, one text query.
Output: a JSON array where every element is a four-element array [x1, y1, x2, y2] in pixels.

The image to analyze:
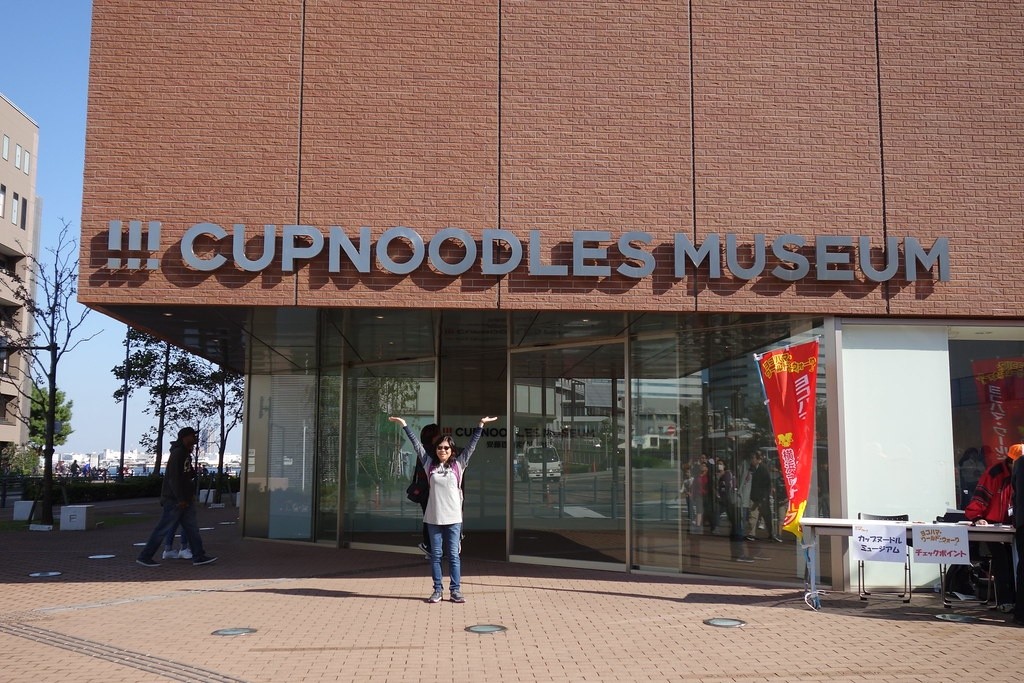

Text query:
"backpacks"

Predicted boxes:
[[718, 470, 737, 496]]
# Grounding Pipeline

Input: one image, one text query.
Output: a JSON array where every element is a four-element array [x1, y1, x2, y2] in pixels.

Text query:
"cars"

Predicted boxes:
[[133, 467, 145, 476], [106, 467, 119, 479], [229, 471, 238, 476], [123, 466, 137, 474]]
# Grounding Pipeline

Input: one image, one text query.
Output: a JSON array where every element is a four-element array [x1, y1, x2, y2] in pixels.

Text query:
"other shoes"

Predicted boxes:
[[745, 535, 755, 541], [418, 543, 431, 556], [192, 555, 218, 566], [428, 590, 443, 602], [424, 554, 431, 560], [450, 589, 465, 603], [999, 603, 1016, 613], [769, 537, 783, 543], [731, 556, 754, 563], [711, 528, 720, 533], [136, 557, 160, 567]]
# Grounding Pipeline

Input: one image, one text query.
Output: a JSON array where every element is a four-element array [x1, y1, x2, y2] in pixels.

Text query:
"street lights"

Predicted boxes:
[[565, 425, 571, 453]]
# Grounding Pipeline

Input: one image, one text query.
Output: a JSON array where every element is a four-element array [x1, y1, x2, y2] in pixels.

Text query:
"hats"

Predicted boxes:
[[178, 427, 201, 440], [1006, 444, 1024, 460]]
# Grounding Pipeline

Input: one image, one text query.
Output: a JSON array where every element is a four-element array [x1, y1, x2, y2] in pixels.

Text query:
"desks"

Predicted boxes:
[[796, 517, 1016, 606]]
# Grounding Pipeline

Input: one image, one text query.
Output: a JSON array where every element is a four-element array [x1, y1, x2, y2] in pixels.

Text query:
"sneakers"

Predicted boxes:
[[178, 547, 193, 559], [162, 551, 179, 559]]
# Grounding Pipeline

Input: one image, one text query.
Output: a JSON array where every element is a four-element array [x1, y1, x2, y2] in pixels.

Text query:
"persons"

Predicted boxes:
[[677, 449, 829, 562], [54, 460, 80, 478], [387, 416, 498, 603], [418, 423, 443, 561], [197, 462, 230, 475], [956, 443, 1024, 625], [161, 444, 200, 561], [135, 427, 218, 567]]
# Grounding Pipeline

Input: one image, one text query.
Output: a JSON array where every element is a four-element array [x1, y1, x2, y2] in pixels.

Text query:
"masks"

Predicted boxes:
[[716, 464, 725, 470]]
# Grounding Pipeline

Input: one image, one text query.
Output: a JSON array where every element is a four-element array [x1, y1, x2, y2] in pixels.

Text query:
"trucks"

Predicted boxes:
[[631, 434, 677, 449]]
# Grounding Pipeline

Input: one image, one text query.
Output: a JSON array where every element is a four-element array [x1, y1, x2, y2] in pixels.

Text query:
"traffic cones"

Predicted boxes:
[[592, 463, 596, 472], [375, 486, 381, 510], [547, 481, 554, 503]]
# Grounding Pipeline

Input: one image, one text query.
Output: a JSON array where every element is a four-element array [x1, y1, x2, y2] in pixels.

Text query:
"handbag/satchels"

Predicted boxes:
[[406, 483, 429, 504]]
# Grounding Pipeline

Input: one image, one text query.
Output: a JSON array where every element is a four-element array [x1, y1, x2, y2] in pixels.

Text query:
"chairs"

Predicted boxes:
[[937, 512, 999, 614], [856, 513, 914, 607]]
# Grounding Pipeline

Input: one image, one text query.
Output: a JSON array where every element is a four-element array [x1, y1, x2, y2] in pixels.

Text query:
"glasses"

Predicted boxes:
[[436, 445, 451, 450]]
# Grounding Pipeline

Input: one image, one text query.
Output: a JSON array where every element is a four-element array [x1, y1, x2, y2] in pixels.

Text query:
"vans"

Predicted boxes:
[[521, 446, 562, 481]]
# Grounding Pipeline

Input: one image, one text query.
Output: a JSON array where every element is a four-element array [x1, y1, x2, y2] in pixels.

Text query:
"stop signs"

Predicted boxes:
[[668, 426, 675, 434]]
